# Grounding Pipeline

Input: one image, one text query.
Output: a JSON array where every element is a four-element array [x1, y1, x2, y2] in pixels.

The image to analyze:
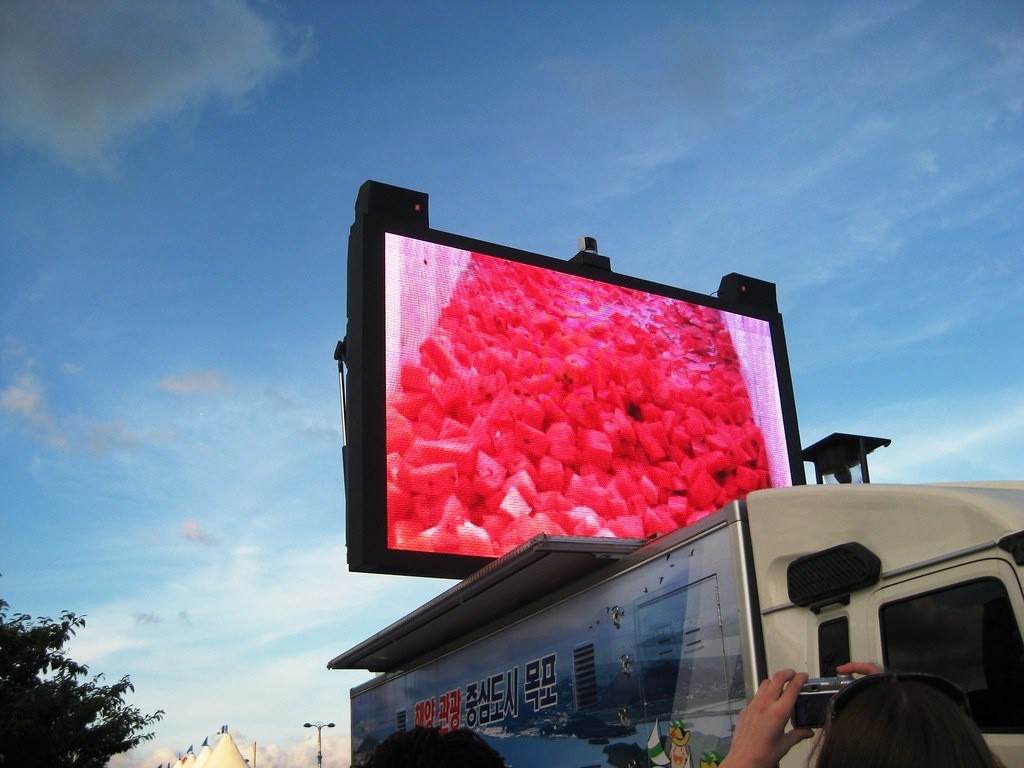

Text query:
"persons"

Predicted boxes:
[[718, 662, 1001, 768]]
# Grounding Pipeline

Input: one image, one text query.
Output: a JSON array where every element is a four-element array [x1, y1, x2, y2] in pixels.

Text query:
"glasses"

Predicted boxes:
[[828, 673, 972, 720]]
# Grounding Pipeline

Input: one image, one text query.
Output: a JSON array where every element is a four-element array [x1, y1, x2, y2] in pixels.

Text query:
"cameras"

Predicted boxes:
[[790, 673, 856, 729]]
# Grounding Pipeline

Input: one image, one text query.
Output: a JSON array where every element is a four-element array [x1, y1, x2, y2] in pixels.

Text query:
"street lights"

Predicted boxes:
[[304, 720, 335, 768]]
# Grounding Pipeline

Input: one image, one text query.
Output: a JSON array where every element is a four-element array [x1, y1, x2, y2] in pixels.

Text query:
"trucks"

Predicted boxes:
[[351, 479, 1024, 768]]
